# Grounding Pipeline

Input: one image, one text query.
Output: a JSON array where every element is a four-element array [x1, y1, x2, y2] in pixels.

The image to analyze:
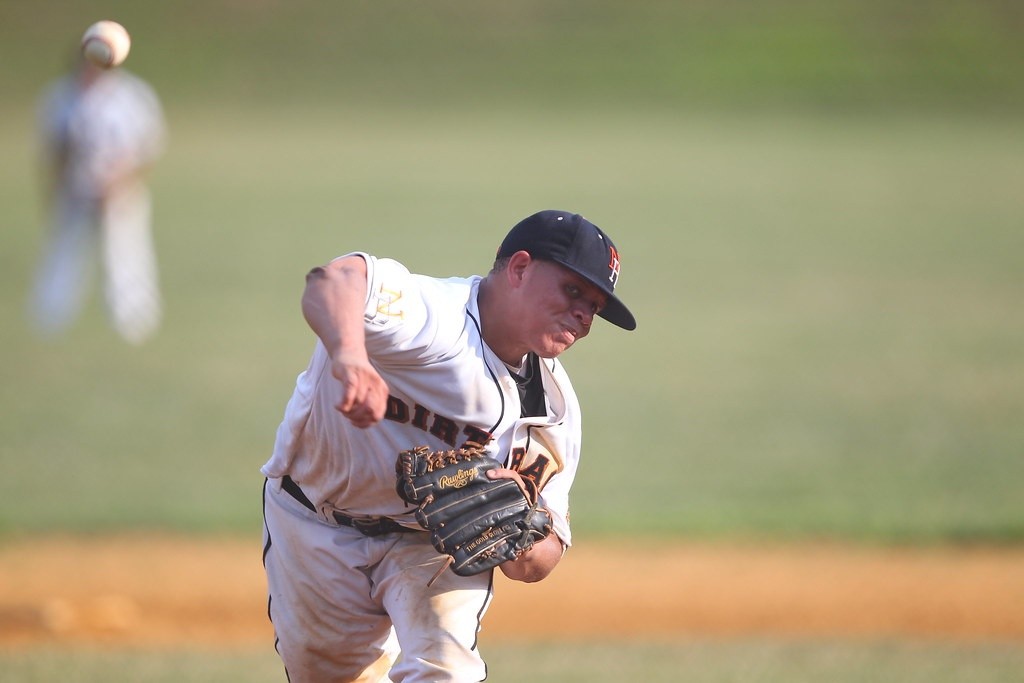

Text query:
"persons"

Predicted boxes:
[[262, 209, 636, 683], [34, 66, 165, 334]]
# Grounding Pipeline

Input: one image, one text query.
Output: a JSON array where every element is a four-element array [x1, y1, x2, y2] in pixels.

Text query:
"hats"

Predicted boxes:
[[496, 209, 637, 331]]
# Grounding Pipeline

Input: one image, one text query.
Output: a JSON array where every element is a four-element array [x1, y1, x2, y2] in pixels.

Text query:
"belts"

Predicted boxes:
[[280, 475, 422, 537]]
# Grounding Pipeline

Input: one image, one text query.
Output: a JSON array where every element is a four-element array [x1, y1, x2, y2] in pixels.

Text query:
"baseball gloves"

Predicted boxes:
[[394, 443, 554, 579]]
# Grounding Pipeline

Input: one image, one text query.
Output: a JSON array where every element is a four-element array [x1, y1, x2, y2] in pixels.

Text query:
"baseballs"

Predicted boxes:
[[80, 19, 133, 71]]
